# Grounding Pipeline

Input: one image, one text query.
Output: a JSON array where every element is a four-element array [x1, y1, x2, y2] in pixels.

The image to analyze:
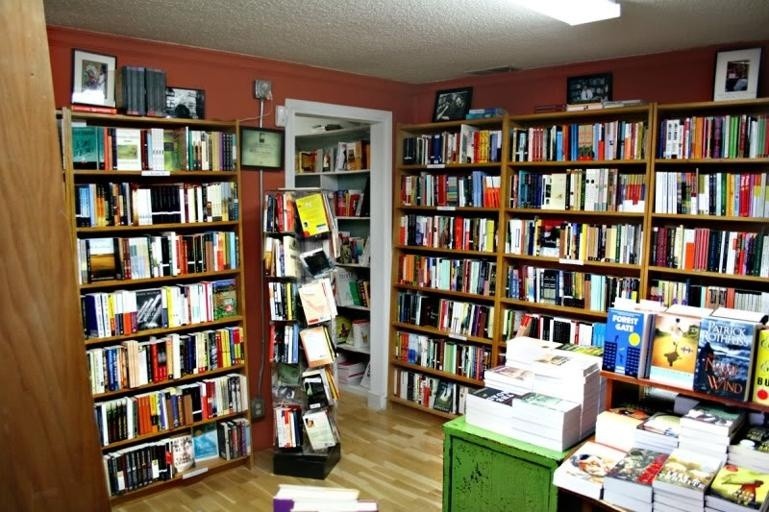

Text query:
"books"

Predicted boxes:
[[272, 483, 377, 511], [261, 190, 342, 452], [74, 182, 239, 227], [95, 374, 252, 496], [80, 279, 246, 395], [467, 297, 768, 512], [399, 124, 502, 253], [115, 67, 206, 118], [503, 99, 769, 349], [56, 119, 237, 171], [76, 231, 240, 284], [393, 254, 496, 415], [297, 141, 370, 387], [466, 108, 507, 119]]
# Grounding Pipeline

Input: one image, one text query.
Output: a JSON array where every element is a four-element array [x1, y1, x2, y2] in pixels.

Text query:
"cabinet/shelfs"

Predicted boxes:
[[262, 184, 341, 479], [296, 127, 369, 398], [497, 101, 654, 376], [646, 98, 769, 374], [395, 119, 505, 432], [63, 112, 254, 497], [548, 360, 768, 511]]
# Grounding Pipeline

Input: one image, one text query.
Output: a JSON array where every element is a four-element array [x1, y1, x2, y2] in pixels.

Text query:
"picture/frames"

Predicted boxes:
[[431, 85, 473, 122], [711, 46, 762, 101], [70, 46, 118, 109], [565, 70, 614, 104]]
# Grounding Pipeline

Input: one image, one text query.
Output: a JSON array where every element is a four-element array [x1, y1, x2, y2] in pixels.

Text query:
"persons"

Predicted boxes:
[[581, 84, 592, 101], [83, 66, 106, 97], [436, 93, 466, 121]]
